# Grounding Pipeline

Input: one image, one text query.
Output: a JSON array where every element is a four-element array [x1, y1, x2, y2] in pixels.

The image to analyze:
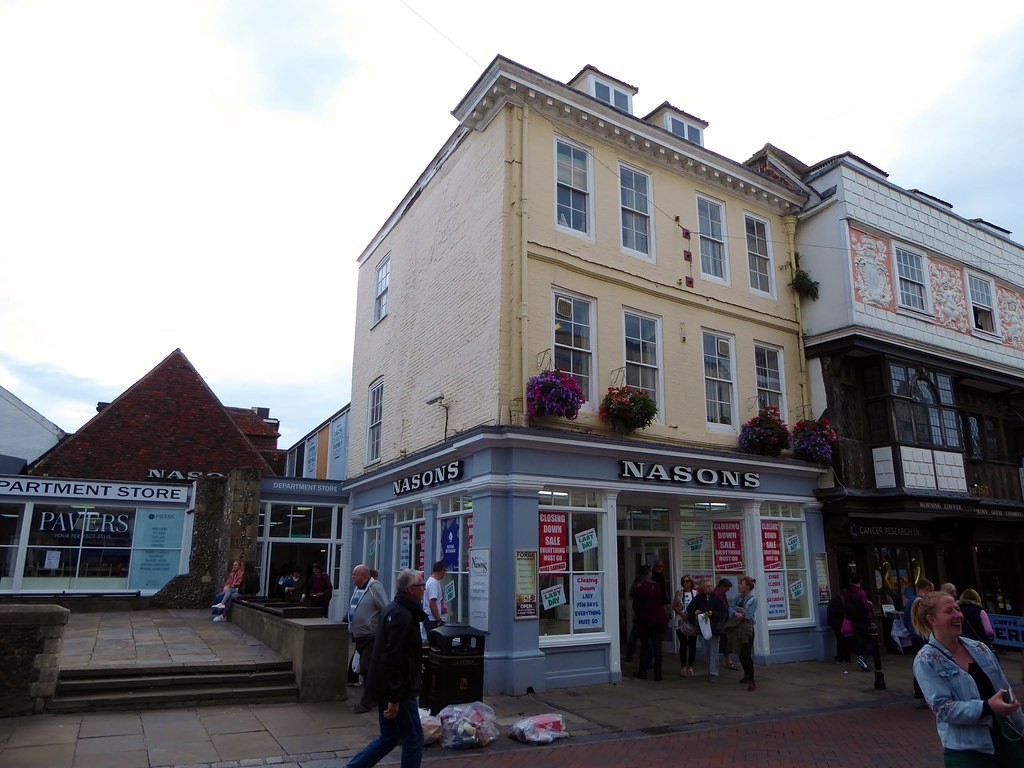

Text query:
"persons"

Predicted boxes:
[[651, 559, 665, 591], [687, 582, 736, 677], [940, 583, 956, 601], [285, 566, 306, 606], [211, 561, 260, 623], [342, 569, 428, 767], [673, 575, 699, 676], [342, 565, 389, 687], [633, 586, 671, 682], [309, 563, 332, 618], [826, 588, 851, 663], [625, 565, 656, 669], [903, 578, 934, 698], [422, 562, 447, 641], [707, 578, 732, 683], [957, 589, 994, 652], [729, 577, 757, 690], [844, 572, 873, 671], [911, 592, 1024, 768]]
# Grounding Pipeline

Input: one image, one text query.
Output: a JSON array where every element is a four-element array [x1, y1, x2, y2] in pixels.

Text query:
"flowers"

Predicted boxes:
[[525, 368, 586, 421], [792, 417, 846, 466], [597, 385, 659, 435], [738, 405, 795, 457]]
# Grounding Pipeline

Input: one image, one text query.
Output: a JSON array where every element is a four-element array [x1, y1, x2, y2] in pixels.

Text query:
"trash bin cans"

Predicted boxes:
[[426, 621, 486, 716]]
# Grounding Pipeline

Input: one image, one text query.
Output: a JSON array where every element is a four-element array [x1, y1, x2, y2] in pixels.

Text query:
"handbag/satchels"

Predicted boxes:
[[698, 613, 712, 640], [989, 645, 1000, 662], [351, 650, 360, 673], [681, 622, 697, 636]]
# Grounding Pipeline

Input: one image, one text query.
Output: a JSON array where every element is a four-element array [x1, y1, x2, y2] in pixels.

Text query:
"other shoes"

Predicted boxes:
[[687, 666, 694, 677], [350, 704, 370, 713], [709, 675, 721, 683], [747, 682, 756, 691], [855, 656, 873, 672], [739, 678, 749, 683], [624, 657, 633, 663], [727, 663, 739, 670], [211, 603, 226, 609], [213, 614, 227, 623], [347, 682, 363, 687], [632, 672, 647, 680], [679, 666, 688, 677]]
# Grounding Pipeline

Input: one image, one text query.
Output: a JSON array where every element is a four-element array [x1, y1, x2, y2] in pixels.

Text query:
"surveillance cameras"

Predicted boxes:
[[426, 392, 444, 406]]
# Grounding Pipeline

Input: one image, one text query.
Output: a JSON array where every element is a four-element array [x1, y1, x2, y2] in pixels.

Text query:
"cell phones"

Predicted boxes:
[[1002, 687, 1015, 704]]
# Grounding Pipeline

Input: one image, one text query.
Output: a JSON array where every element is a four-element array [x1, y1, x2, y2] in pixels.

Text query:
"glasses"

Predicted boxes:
[[681, 580, 690, 583], [352, 572, 362, 577], [411, 583, 427, 589]]
